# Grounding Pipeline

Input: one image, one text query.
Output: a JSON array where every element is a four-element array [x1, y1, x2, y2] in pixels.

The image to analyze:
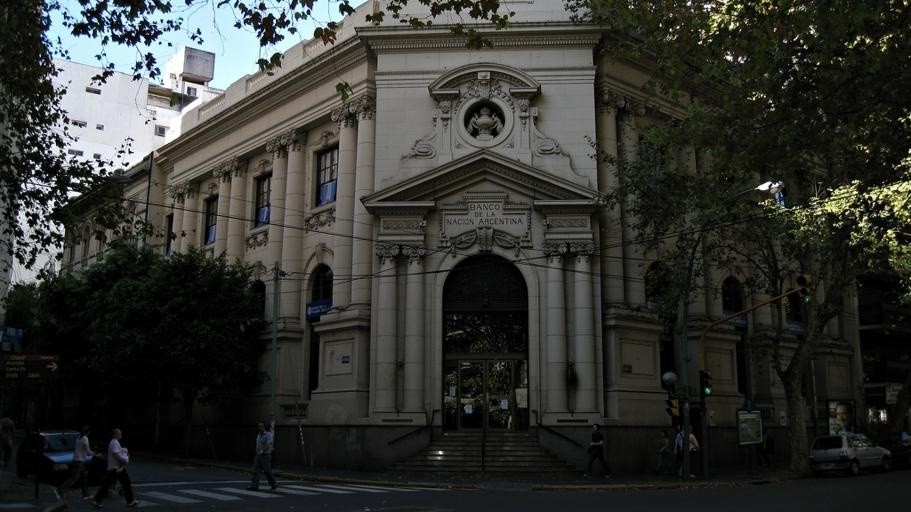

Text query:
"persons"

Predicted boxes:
[[674, 425, 684, 476], [657, 430, 671, 473], [53, 425, 103, 501], [1, 409, 17, 469], [582, 423, 615, 479], [249, 421, 280, 490], [90, 427, 142, 505], [836, 401, 854, 432], [689, 426, 703, 480]]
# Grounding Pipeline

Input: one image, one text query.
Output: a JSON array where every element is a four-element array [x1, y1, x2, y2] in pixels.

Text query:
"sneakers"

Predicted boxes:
[[246, 484, 280, 491], [54, 489, 140, 508]]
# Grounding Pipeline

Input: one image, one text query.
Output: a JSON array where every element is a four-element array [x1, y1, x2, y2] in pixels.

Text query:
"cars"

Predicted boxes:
[[808, 434, 891, 475], [893, 434, 910, 469], [29, 428, 97, 484]]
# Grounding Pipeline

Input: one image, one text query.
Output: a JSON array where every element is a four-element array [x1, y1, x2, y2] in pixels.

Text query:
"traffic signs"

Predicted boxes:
[[0, 353, 60, 380]]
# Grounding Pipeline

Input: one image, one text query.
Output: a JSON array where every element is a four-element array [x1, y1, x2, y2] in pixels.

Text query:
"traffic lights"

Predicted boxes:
[[665, 397, 679, 416], [798, 273, 814, 304], [702, 370, 712, 395]]
